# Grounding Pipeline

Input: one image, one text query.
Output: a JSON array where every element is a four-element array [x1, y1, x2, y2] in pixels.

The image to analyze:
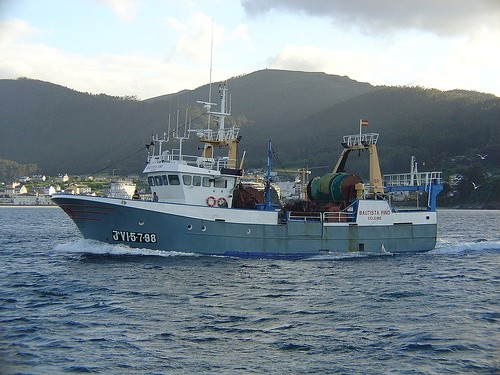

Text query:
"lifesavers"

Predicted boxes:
[[217, 197, 227, 206], [206, 195, 217, 207]]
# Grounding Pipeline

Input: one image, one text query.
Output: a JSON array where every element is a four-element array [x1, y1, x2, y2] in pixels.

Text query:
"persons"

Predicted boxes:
[[153, 192, 158, 202], [132, 190, 140, 201]]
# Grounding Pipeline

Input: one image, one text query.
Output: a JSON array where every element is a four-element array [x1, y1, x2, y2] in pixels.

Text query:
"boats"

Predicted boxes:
[[48, 22, 445, 257]]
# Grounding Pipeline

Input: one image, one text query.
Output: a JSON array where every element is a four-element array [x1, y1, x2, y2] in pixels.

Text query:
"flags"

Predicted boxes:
[[362, 120, 368, 126]]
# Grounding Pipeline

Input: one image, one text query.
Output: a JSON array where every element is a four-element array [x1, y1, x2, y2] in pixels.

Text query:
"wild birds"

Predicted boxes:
[[476, 153, 488, 160], [470, 181, 482, 190]]
